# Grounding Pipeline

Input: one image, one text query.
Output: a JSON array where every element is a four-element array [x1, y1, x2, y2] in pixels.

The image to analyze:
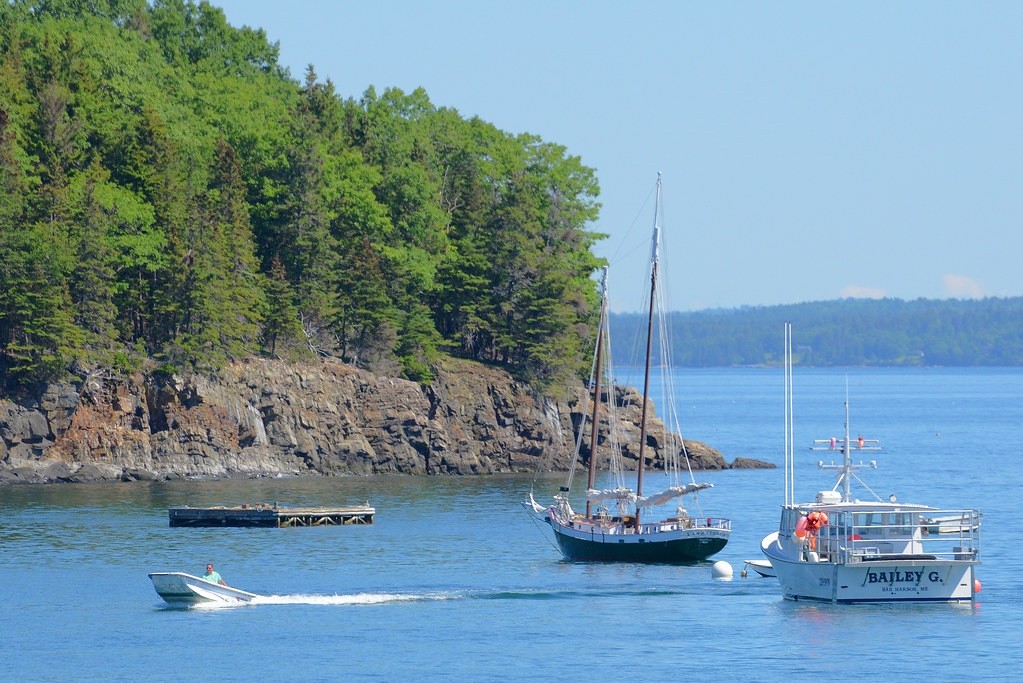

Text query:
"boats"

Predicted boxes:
[[742, 321, 985, 604], [148, 573, 259, 605]]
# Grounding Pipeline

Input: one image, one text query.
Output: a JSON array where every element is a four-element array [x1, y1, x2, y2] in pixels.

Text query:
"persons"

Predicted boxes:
[[201, 563, 227, 587]]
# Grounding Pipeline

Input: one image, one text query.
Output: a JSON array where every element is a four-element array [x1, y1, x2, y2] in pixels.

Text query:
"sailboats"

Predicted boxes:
[[522, 171, 733, 564]]
